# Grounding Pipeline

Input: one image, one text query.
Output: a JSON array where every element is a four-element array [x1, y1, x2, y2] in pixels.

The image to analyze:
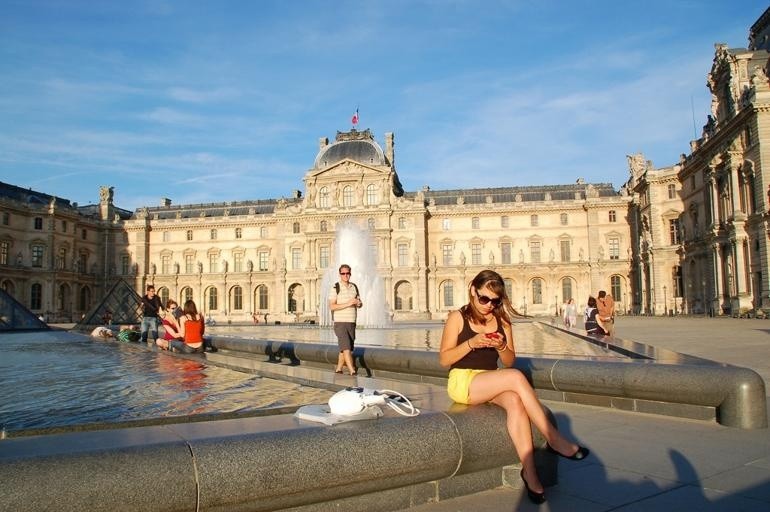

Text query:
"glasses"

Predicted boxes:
[[340, 272, 350, 275], [475, 289, 501, 307]]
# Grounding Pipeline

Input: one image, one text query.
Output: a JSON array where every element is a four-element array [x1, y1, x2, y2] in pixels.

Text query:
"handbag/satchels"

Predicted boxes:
[[329, 387, 420, 417]]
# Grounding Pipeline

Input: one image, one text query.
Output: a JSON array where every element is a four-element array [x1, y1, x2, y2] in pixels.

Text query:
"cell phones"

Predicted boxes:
[[485, 333, 500, 340]]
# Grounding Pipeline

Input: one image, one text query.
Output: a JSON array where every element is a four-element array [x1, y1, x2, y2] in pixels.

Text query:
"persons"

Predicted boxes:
[[566, 298, 577, 328], [439, 270, 589, 505], [156, 300, 183, 351], [169, 300, 205, 354], [327, 264, 363, 376], [139, 285, 165, 342], [561, 297, 570, 326], [584, 297, 611, 336], [595, 291, 616, 336]]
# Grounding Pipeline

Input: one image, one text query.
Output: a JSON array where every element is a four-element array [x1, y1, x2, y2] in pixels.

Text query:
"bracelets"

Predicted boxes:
[[467, 338, 476, 352], [497, 344, 507, 353]]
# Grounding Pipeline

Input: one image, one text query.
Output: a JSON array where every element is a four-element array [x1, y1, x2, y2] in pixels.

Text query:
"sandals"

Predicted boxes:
[[520, 468, 545, 503], [546, 441, 589, 460]]
